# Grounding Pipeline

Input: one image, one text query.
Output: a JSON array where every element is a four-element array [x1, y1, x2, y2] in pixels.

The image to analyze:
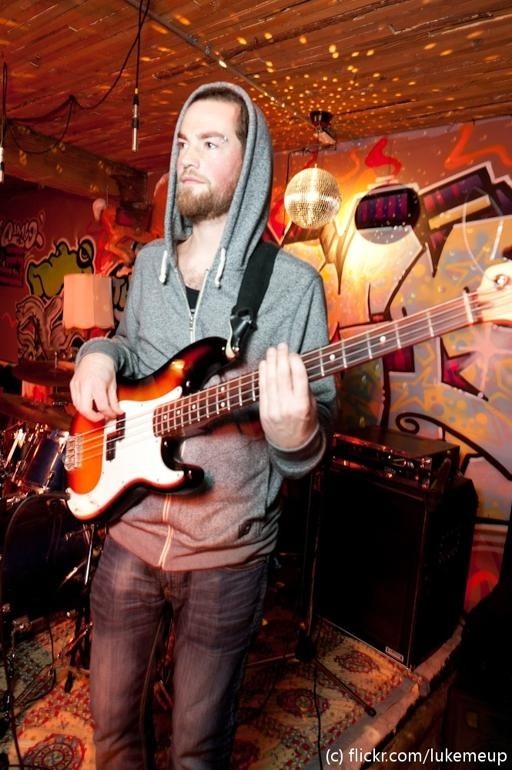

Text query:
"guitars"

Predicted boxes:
[[64, 260, 511, 523]]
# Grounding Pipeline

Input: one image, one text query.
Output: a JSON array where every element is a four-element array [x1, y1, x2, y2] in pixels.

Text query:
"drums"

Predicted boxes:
[[1, 494, 95, 620], [0, 393, 73, 494]]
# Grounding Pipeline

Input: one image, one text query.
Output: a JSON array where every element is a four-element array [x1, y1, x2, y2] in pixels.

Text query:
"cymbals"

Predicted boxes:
[[15, 361, 77, 387]]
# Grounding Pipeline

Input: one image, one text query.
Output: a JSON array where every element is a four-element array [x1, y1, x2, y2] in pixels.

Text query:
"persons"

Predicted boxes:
[[70, 80, 336, 769]]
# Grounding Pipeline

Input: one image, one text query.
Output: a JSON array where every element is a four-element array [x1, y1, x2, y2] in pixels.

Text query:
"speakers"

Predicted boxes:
[[316, 467, 479, 672]]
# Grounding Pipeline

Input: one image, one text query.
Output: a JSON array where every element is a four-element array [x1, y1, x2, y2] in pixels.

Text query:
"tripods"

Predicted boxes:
[[64, 523, 109, 693], [242, 468, 378, 717]]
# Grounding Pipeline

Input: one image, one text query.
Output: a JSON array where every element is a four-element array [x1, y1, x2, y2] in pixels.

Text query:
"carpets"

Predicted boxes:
[[1, 604, 428, 770]]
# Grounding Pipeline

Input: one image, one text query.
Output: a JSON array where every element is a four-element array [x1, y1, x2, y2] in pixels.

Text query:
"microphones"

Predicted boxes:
[[132, 88, 139, 153]]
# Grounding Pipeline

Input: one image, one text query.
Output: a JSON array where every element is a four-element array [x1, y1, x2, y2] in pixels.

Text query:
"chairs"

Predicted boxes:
[[1, 496, 96, 691]]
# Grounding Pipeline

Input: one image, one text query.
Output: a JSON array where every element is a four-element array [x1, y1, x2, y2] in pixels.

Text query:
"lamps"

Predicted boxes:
[[284, 111, 341, 230], [63, 272, 115, 340]]
